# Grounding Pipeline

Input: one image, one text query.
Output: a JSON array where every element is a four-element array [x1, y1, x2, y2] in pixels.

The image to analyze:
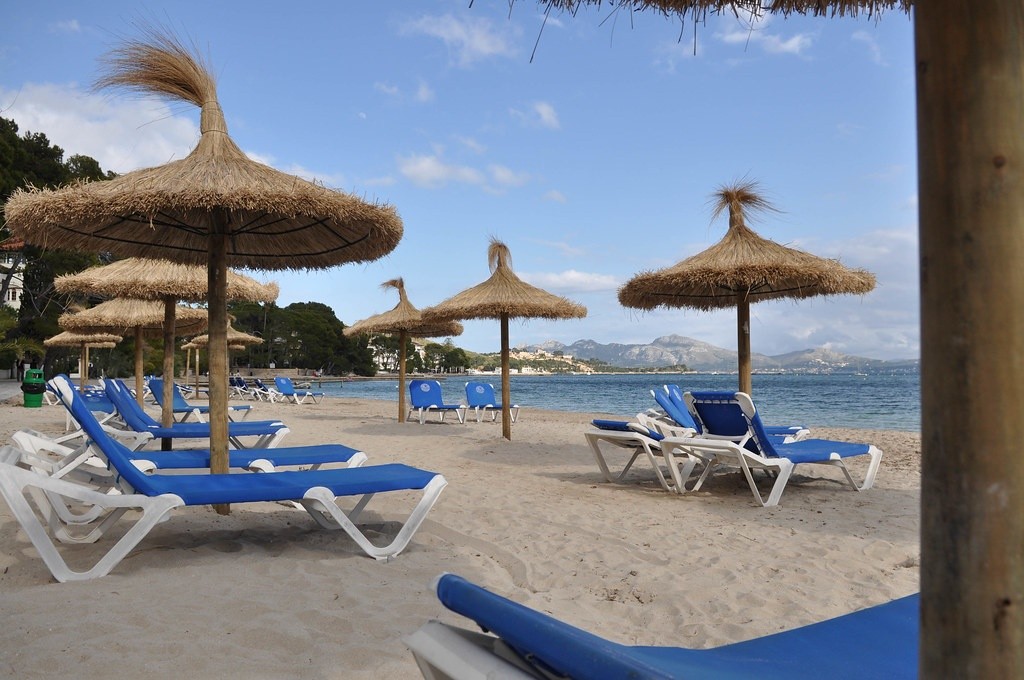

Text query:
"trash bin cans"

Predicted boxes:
[[22, 369, 46, 408]]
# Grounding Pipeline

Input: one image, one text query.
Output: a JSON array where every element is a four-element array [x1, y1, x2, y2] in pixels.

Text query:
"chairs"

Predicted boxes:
[[0, 360, 518, 584], [404, 572, 919, 679], [583, 382, 883, 508]]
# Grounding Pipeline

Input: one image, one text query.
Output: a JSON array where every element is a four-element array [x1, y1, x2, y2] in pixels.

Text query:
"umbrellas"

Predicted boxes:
[[341, 279, 462, 419], [422, 241, 586, 440], [618, 183, 876, 394], [5, 29, 402, 515]]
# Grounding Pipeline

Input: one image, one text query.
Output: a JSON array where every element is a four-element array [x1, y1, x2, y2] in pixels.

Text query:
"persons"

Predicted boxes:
[[30, 358, 38, 378], [20, 360, 25, 381]]
